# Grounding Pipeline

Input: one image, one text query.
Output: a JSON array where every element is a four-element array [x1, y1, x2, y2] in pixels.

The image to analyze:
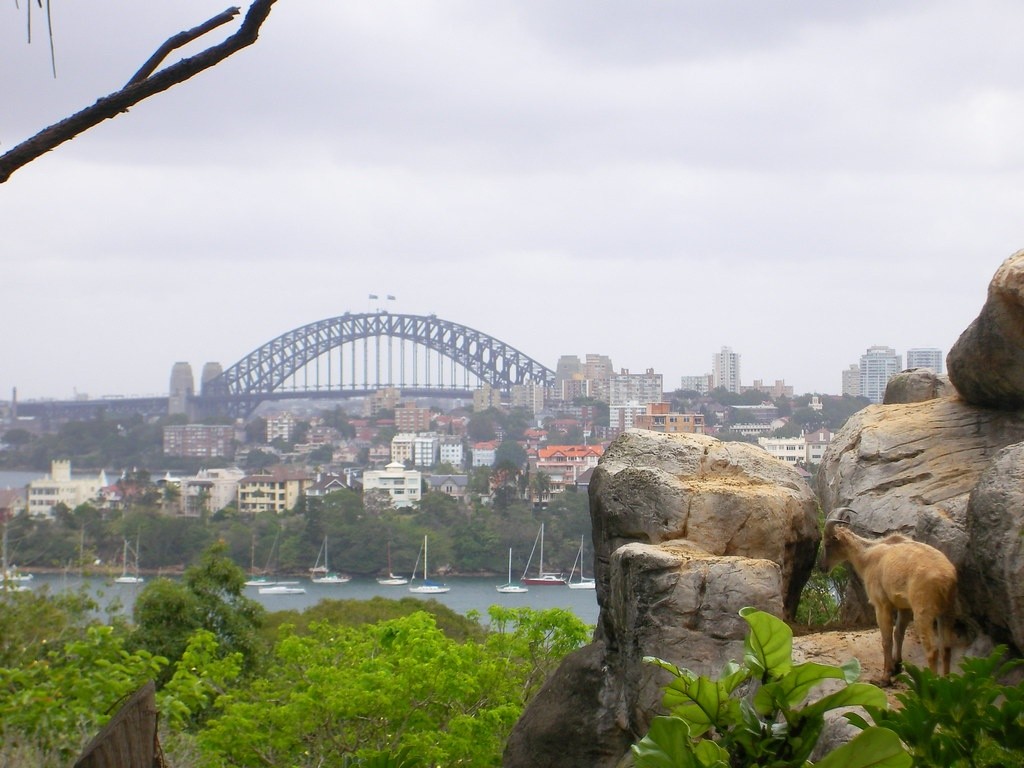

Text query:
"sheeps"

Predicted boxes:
[[818, 507, 958, 689]]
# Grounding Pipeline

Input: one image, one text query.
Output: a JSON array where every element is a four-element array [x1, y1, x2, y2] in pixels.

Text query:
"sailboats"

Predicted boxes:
[[308, 534, 350, 584], [114, 541, 143, 584], [567, 534, 596, 589], [258, 525, 305, 595], [521, 522, 566, 585], [376, 539, 408, 585], [409, 536, 451, 594], [495, 547, 529, 594], [243, 533, 273, 585], [0, 565, 34, 580], [0, 514, 33, 594]]
